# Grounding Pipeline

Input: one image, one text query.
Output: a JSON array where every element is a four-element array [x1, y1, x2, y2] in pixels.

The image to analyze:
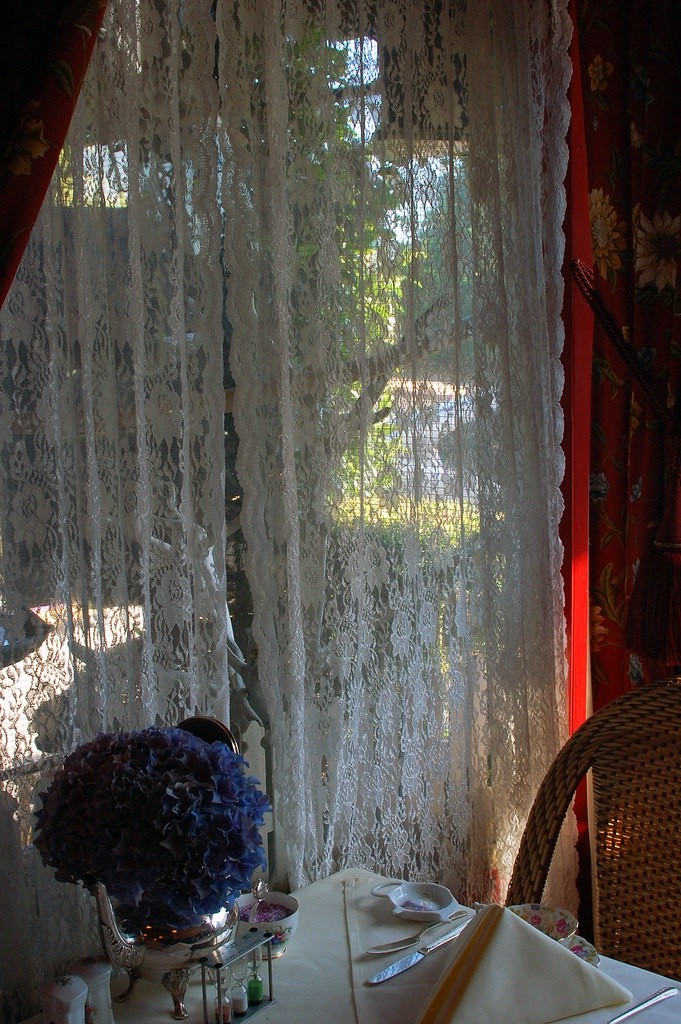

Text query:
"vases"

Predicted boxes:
[[93, 882, 240, 1019]]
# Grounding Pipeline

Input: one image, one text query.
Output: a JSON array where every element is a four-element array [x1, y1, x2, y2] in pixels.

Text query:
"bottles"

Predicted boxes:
[[39, 976, 88, 1024], [73, 956, 114, 1023]]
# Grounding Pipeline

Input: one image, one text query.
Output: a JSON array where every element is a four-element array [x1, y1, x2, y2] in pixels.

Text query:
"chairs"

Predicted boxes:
[[505, 677, 681, 984]]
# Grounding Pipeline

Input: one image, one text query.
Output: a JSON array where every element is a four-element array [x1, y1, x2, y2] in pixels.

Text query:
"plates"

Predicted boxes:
[[558, 934, 600, 967]]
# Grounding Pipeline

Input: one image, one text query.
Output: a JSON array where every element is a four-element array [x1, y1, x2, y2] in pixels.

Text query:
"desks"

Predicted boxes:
[[24, 868, 681, 1024]]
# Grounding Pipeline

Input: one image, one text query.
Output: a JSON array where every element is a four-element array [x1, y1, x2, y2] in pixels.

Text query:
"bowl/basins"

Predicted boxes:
[[370, 879, 459, 921], [234, 892, 300, 961], [508, 904, 578, 942]]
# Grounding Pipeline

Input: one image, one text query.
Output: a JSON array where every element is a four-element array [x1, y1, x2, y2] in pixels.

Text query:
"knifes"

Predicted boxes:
[[366, 910, 468, 953], [368, 916, 473, 983]]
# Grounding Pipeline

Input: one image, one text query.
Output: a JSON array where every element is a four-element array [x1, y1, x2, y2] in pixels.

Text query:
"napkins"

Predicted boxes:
[[345, 878, 632, 1024]]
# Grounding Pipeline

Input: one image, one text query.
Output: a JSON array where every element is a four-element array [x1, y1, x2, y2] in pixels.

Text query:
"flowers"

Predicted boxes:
[[32, 728, 274, 933]]
